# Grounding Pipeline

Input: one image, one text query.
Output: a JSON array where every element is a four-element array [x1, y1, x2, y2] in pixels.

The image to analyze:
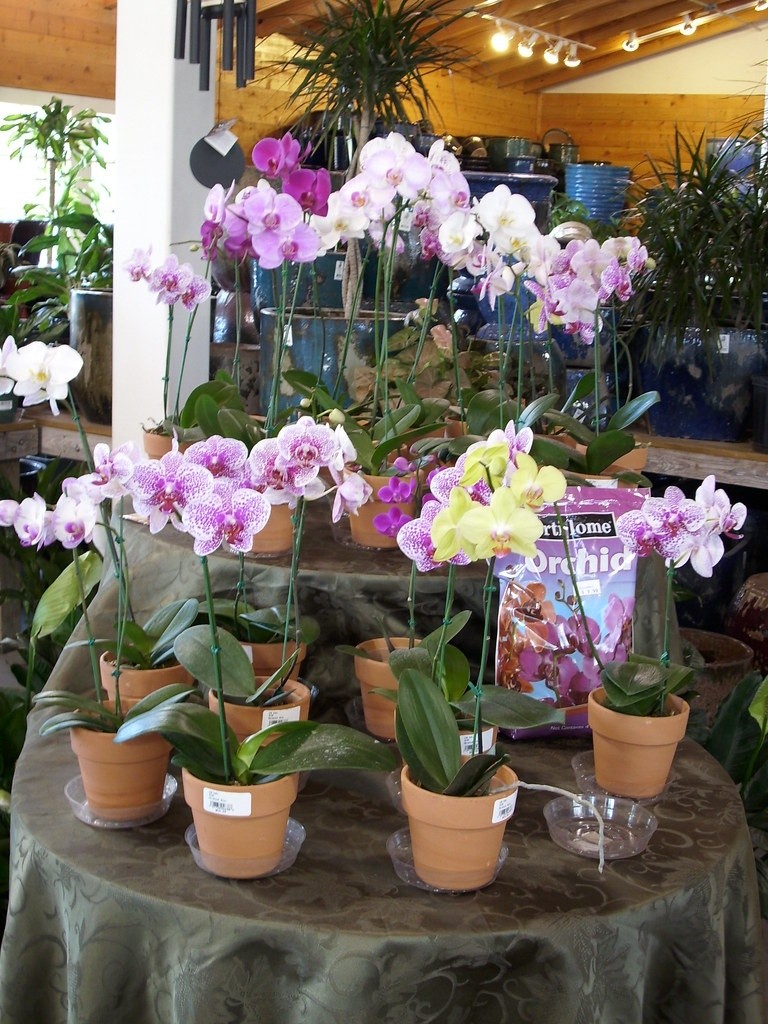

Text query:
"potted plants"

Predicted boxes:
[[0, 96, 112, 424], [609, 60, 767, 454], [248, 0, 480, 422]]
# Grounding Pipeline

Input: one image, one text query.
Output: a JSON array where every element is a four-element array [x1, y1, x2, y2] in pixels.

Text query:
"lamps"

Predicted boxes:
[[680, 10, 699, 36], [563, 42, 581, 67], [513, 31, 539, 57], [621, 28, 639, 52], [544, 35, 565, 64], [491, 28, 518, 52]]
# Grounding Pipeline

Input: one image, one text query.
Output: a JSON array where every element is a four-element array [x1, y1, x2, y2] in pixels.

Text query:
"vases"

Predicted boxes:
[[181, 743, 300, 876], [401, 757, 519, 891], [208, 674, 310, 747], [454, 712, 499, 766], [69, 699, 175, 821], [588, 687, 690, 799], [100, 648, 194, 700], [227, 503, 292, 560], [677, 625, 754, 728], [236, 639, 306, 682], [538, 432, 650, 489], [344, 466, 424, 548], [354, 637, 421, 741]]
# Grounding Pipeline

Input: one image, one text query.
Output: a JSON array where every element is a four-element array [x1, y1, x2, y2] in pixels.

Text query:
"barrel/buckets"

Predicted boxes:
[[457, 136, 631, 229]]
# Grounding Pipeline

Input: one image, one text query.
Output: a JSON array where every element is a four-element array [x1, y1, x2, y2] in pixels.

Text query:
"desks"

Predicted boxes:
[[638, 427, 768, 489], [0, 419, 41, 491], [23, 410, 112, 463]]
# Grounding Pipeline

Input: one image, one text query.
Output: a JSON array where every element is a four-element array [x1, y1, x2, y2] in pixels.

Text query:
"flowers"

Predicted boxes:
[[0, 126, 746, 797]]
